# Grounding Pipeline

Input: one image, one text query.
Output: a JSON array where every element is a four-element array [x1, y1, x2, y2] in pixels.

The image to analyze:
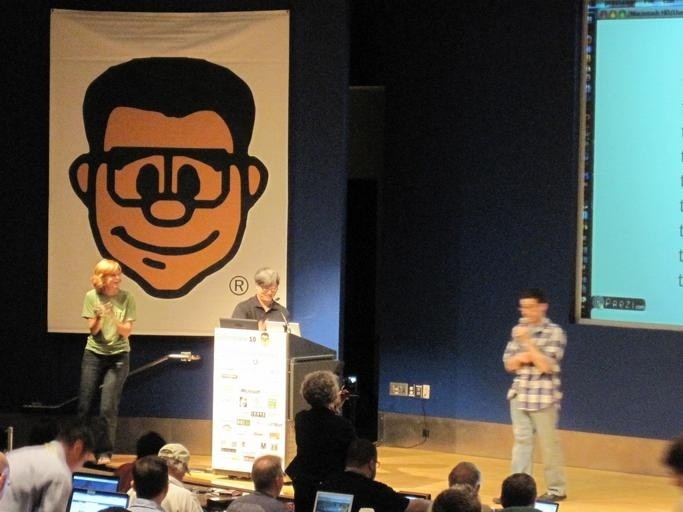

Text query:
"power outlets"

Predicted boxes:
[[422, 384, 430, 400]]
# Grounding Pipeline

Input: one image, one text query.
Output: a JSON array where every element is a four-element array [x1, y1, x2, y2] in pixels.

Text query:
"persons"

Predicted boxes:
[[283, 371, 360, 512], [228, 455, 294, 512], [232, 267, 293, 324], [492, 288, 568, 505], [659, 437, 683, 494], [1, 424, 96, 512], [117, 431, 204, 512], [431, 462, 537, 511], [76, 259, 137, 454], [324, 441, 430, 512]]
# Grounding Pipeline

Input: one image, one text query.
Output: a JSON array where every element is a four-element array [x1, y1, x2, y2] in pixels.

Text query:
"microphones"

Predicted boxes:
[[518, 317, 528, 327], [275, 297, 291, 333]]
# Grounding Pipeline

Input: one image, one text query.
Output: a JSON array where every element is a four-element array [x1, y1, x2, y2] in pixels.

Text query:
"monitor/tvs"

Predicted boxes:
[[400, 491, 431, 501], [220, 318, 259, 330]]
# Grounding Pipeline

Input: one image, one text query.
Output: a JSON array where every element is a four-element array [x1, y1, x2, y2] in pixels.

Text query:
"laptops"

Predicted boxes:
[[313, 490, 354, 512], [72, 472, 120, 492], [66, 488, 130, 512], [535, 500, 559, 512]]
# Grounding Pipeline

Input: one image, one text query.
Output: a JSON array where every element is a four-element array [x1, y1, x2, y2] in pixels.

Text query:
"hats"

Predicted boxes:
[[158, 443, 191, 477]]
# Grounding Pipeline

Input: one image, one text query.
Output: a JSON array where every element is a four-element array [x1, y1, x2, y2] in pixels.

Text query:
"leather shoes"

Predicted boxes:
[[535, 492, 566, 503]]
